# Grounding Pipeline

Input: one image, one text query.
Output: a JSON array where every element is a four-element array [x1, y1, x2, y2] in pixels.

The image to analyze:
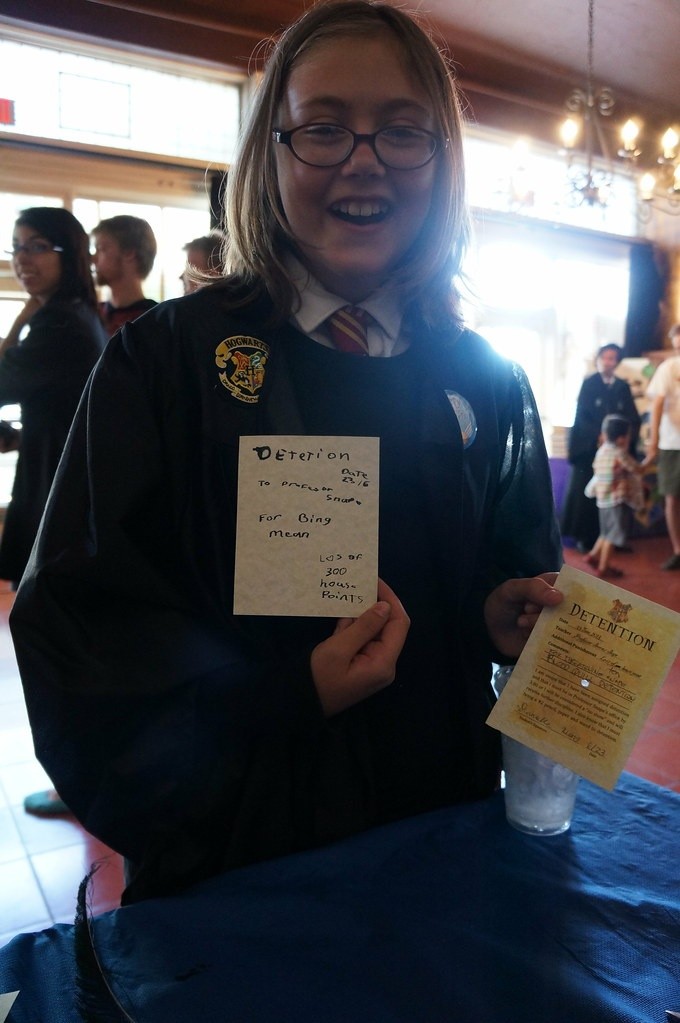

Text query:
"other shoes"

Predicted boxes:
[[594, 563, 623, 577], [583, 555, 599, 569], [661, 555, 680, 571], [22, 791, 77, 819]]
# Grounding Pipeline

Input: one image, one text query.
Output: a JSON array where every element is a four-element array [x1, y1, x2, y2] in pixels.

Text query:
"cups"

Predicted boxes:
[[494, 663, 576, 836]]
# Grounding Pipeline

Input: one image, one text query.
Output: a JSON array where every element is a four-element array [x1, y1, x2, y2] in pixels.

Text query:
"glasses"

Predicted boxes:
[[269, 122, 444, 170], [4, 239, 67, 255]]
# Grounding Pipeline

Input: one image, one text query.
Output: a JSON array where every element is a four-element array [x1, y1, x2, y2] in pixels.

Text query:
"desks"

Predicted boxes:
[[0, 764, 680, 1023]]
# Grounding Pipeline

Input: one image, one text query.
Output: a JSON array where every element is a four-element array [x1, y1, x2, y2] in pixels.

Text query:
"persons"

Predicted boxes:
[[91, 216, 158, 338], [6, 0, 565, 906], [642, 324, 680, 571], [0, 207, 113, 813], [558, 342, 641, 552], [583, 415, 658, 575], [179, 230, 225, 293]]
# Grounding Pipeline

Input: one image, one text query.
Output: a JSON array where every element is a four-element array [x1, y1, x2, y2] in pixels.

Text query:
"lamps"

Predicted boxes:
[[502, 0, 680, 231]]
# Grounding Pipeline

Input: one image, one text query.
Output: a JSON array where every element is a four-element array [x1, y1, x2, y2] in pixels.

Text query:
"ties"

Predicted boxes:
[[323, 304, 376, 359]]
